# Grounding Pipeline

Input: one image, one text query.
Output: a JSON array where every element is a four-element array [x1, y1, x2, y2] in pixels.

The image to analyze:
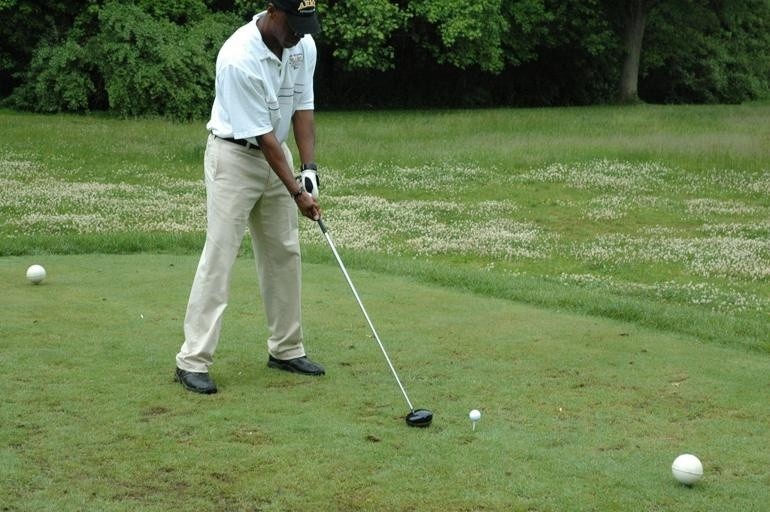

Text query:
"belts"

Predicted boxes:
[[222, 136, 263, 150]]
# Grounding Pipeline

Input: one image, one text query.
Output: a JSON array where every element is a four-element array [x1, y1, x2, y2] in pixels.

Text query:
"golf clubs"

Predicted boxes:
[[315, 218, 434, 426]]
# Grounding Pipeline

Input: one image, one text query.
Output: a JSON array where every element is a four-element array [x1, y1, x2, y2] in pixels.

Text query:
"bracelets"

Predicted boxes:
[[291, 186, 305, 200], [300, 163, 317, 172]]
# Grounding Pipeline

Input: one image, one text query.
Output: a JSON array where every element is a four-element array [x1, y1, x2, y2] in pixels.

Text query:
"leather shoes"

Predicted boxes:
[[267, 355, 325, 375], [175, 365, 218, 393]]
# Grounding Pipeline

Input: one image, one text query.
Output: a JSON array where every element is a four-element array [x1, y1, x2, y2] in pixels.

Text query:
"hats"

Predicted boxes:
[[274, 0, 323, 37]]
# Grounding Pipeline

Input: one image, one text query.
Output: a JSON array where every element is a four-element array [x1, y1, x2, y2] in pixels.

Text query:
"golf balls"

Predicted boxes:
[[672, 455, 703, 485], [469, 410, 482, 419], [27, 264, 45, 285]]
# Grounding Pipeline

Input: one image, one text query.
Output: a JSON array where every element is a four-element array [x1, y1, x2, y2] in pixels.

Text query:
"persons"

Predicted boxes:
[[172, 1, 330, 395]]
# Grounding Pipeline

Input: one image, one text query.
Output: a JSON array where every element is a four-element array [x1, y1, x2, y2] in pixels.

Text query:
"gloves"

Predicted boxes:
[[295, 170, 320, 202]]
